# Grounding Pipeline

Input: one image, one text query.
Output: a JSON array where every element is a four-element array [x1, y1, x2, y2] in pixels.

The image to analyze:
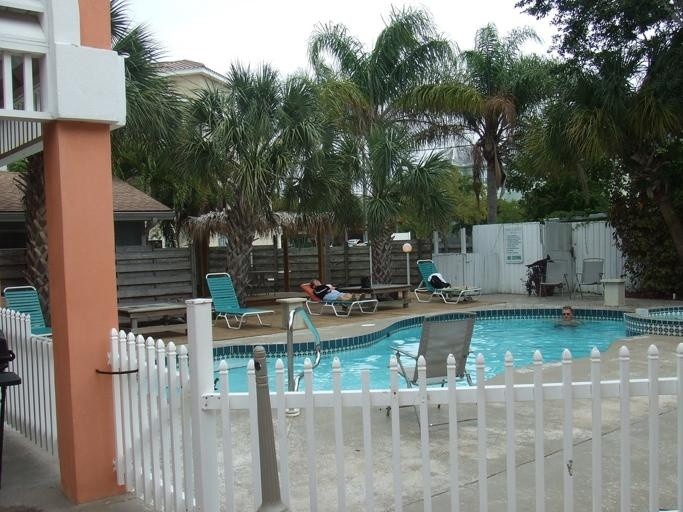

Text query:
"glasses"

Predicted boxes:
[[562, 313, 571, 316]]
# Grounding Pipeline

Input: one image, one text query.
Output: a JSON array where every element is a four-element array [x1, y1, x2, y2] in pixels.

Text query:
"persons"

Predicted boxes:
[[310, 278, 366, 301], [553, 305, 582, 330]]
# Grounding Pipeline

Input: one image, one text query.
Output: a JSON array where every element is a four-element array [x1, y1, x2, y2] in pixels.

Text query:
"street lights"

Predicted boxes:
[[402, 243, 412, 294]]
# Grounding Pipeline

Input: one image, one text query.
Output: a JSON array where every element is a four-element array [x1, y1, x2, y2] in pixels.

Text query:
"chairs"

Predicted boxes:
[[386, 311, 486, 430], [300, 283, 380, 316], [538, 257, 606, 300], [414, 259, 482, 305], [205, 272, 274, 329], [4, 286, 52, 338]]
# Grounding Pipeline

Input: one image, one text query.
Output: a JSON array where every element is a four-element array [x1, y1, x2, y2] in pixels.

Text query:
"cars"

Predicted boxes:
[[347, 239, 362, 247]]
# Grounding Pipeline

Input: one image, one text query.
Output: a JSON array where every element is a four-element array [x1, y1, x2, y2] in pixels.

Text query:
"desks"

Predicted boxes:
[[338, 283, 412, 311], [599, 278, 626, 307], [276, 297, 308, 329], [118, 302, 187, 337]]
